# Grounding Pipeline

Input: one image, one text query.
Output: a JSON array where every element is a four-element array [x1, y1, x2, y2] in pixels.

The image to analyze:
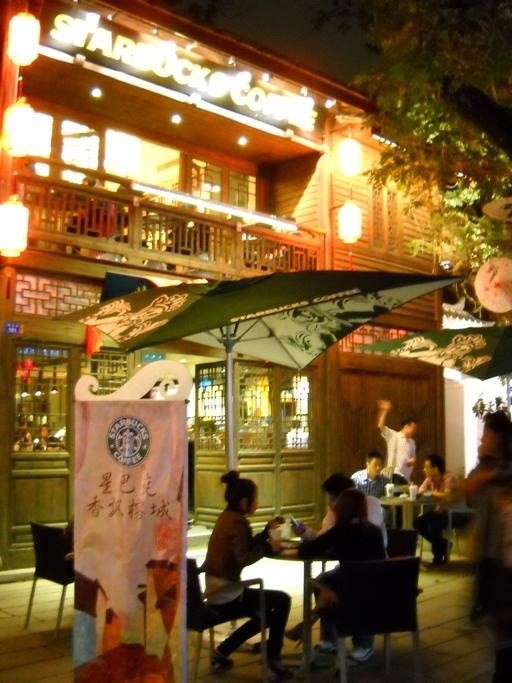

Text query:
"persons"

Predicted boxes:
[[412, 410, 512, 682], [377, 400, 417, 484], [289, 472, 390, 663], [349, 450, 391, 499], [204, 472, 295, 680], [284, 489, 386, 650]]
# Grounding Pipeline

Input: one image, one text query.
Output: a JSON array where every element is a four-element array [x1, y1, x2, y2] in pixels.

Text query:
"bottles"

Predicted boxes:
[[388, 467, 393, 482]]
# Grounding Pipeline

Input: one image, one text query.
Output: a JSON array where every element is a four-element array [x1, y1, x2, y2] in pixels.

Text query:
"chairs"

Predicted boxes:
[[185, 559, 268, 681], [319, 557, 422, 679], [384, 528, 417, 559], [416, 494, 471, 562], [24, 521, 74, 639]]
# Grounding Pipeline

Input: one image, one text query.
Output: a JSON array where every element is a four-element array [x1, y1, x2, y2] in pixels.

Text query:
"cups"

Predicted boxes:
[[268, 528, 281, 539], [409, 484, 418, 500], [385, 483, 394, 496]]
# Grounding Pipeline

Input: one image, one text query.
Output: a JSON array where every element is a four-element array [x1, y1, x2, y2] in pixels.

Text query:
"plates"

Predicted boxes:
[[268, 539, 302, 549]]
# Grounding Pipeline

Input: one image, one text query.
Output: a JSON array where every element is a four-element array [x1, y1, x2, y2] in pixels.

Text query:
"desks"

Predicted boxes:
[[264, 540, 339, 681], [378, 494, 443, 530]]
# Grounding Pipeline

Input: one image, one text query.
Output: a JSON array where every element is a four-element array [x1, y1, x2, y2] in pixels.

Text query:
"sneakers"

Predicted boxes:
[[314, 640, 337, 654], [350, 647, 374, 661]]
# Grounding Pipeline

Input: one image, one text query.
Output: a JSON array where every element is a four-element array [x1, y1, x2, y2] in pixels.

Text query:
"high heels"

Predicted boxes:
[[285, 623, 304, 649], [213, 650, 234, 668], [259, 657, 292, 678]]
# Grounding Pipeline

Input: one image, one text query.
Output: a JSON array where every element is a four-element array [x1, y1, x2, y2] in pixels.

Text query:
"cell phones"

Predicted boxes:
[[290, 516, 300, 527]]
